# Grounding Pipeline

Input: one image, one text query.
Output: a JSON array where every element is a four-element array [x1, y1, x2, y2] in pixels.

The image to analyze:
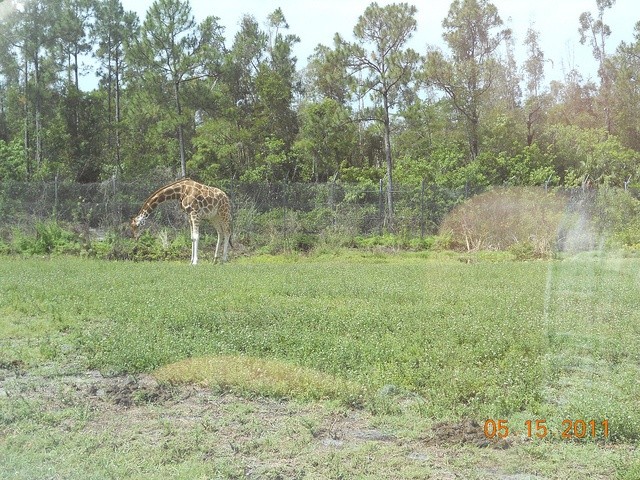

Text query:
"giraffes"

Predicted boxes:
[[129, 177, 234, 266]]
